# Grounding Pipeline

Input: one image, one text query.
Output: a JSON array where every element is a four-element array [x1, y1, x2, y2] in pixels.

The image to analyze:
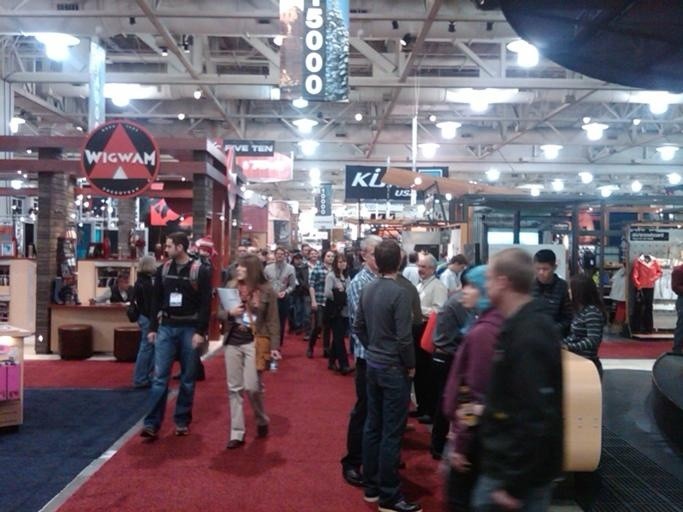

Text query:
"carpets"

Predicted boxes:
[[0, 385, 171, 512]]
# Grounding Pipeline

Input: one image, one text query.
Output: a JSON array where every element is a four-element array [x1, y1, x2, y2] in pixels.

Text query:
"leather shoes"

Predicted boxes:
[[364, 493, 380, 502], [257, 425, 267, 437], [378, 501, 422, 512], [409, 409, 423, 417], [417, 413, 431, 423], [343, 467, 363, 486], [227, 441, 241, 448]]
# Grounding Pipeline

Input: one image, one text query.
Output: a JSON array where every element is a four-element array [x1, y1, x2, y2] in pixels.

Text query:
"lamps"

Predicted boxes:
[[657, 145, 678, 162], [10, 179, 24, 191], [104, 82, 141, 106], [415, 177, 423, 184], [646, 89, 671, 114], [294, 97, 308, 110], [298, 139, 319, 156], [308, 166, 322, 195], [8, 116, 26, 135], [634, 119, 641, 126], [419, 143, 440, 159], [632, 181, 644, 194], [584, 115, 591, 122], [446, 193, 453, 202], [36, 32, 78, 60], [183, 43, 189, 53], [542, 144, 563, 158], [551, 179, 566, 192], [273, 34, 284, 46], [437, 122, 462, 140], [529, 184, 543, 196], [240, 187, 275, 202], [194, 91, 203, 100], [400, 32, 412, 46], [600, 186, 618, 199], [582, 124, 607, 140], [292, 118, 318, 134], [162, 48, 168, 57], [74, 195, 92, 208], [486, 170, 501, 183], [507, 41, 540, 65], [354, 113, 364, 122], [472, 94, 490, 115], [579, 172, 594, 183], [17, 147, 33, 178], [667, 173, 680, 186], [428, 114, 437, 124], [178, 113, 186, 120]]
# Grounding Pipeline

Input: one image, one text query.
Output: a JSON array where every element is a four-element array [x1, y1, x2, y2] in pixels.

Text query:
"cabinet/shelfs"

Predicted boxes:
[[75, 258, 162, 305], [0, 257, 36, 337], [0, 322, 34, 429]]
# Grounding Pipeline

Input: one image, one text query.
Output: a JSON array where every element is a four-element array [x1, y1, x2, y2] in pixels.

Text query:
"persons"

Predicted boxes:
[[630, 253, 662, 334], [215, 253, 281, 448], [669, 263, 683, 354], [530, 249, 572, 337], [224, 243, 365, 375], [139, 230, 214, 438], [565, 275, 605, 377], [340, 235, 563, 511], [186, 233, 218, 268], [130, 254, 159, 391], [88, 272, 135, 304]]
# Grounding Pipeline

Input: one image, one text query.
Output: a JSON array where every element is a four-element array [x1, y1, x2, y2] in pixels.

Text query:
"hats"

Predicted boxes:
[[195, 235, 214, 256]]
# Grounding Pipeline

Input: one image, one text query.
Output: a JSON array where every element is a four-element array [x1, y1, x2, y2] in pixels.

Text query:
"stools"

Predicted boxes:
[[57, 325, 92, 361], [112, 327, 141, 360]]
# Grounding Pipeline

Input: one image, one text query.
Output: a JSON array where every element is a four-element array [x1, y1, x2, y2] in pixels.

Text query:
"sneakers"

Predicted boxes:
[[324, 348, 329, 357], [308, 347, 314, 357], [175, 427, 189, 436], [328, 364, 339, 371], [133, 379, 151, 389], [431, 445, 442, 459], [340, 367, 355, 375], [140, 428, 159, 438]]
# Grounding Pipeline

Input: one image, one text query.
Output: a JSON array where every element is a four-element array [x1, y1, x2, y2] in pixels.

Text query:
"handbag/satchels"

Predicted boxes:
[[254, 336, 270, 371], [420, 311, 437, 354], [187, 349, 204, 382]]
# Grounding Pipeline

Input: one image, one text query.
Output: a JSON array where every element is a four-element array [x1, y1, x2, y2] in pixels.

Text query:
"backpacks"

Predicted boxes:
[[127, 283, 139, 322]]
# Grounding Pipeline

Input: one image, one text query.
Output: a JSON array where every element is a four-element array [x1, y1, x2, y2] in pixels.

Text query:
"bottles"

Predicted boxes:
[[269, 354, 279, 373], [458, 387, 478, 427]]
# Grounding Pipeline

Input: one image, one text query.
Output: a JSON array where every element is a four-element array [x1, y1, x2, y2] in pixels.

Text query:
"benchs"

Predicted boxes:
[[650, 351, 683, 461]]
